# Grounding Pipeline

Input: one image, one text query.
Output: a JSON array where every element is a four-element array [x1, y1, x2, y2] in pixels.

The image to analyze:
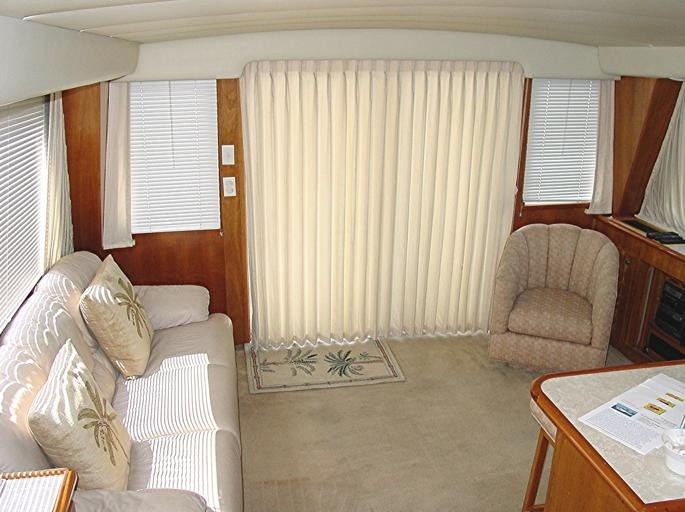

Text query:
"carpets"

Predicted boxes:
[[244, 342, 406, 394]]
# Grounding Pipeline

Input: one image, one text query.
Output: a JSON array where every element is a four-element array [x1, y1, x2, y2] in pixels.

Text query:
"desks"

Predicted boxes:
[[0, 466, 79, 512], [522, 359, 685, 512]]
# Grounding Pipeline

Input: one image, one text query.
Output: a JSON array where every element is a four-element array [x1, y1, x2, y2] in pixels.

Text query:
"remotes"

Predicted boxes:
[[655, 234, 680, 239], [648, 232, 678, 238], [660, 238, 685, 244]]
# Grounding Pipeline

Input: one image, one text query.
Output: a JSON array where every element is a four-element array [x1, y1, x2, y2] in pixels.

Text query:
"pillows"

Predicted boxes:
[[78, 254, 155, 379], [26, 337, 132, 489]]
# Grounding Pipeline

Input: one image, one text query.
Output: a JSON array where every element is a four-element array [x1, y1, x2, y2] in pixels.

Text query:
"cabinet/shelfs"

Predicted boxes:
[[588, 212, 685, 364]]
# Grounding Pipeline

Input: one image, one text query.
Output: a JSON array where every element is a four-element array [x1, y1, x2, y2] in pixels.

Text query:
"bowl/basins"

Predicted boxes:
[[661, 428, 685, 477]]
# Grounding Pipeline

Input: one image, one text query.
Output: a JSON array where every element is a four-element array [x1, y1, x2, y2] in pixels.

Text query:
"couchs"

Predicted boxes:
[[0, 251, 244, 512], [488, 223, 620, 372]]
